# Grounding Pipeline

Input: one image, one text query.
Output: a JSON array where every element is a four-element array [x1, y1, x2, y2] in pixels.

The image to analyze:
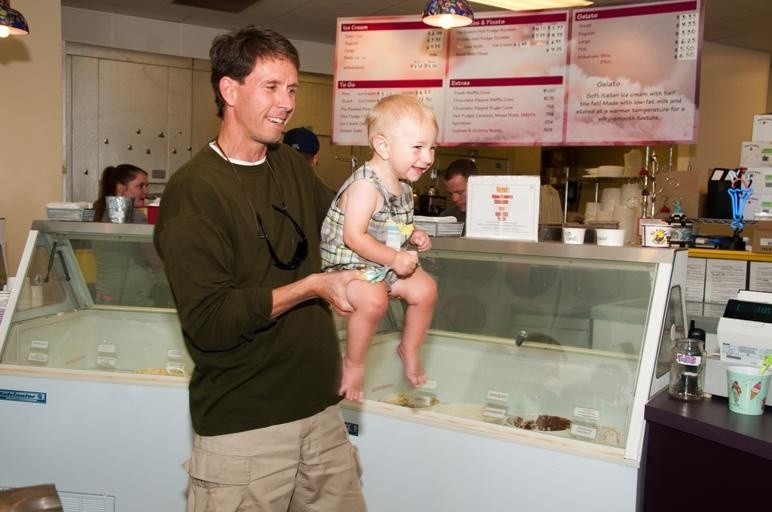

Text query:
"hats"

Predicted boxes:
[[282, 127, 319, 154]]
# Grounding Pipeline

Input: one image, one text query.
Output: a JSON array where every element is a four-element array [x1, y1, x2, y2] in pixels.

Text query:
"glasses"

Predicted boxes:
[[255, 204, 310, 271]]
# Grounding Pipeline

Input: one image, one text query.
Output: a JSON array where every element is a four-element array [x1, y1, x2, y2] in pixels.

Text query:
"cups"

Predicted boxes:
[[103, 195, 134, 226], [726, 365, 771, 416]]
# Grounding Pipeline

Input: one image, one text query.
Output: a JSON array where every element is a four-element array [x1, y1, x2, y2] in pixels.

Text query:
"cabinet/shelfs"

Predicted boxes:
[[563, 145, 657, 240], [0, 221, 772, 510]]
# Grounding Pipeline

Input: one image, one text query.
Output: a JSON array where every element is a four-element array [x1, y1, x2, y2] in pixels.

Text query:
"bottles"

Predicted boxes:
[[667, 338, 707, 403]]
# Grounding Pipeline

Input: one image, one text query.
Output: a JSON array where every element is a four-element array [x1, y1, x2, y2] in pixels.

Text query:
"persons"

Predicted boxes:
[[318, 95, 440, 402], [283, 125, 320, 169], [94, 163, 149, 224], [150, 26, 369, 512], [437, 158, 479, 238]]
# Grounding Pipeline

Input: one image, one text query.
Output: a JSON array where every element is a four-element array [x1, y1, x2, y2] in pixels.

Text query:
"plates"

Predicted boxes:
[[378, 391, 440, 410], [505, 413, 573, 435]]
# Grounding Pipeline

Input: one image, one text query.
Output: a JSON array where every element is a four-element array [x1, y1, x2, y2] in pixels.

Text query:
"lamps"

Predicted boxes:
[[420, 0, 477, 31], [0, 1, 33, 40]]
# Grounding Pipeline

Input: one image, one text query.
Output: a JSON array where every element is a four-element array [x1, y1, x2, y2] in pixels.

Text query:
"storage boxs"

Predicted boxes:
[[739, 114, 771, 219]]
